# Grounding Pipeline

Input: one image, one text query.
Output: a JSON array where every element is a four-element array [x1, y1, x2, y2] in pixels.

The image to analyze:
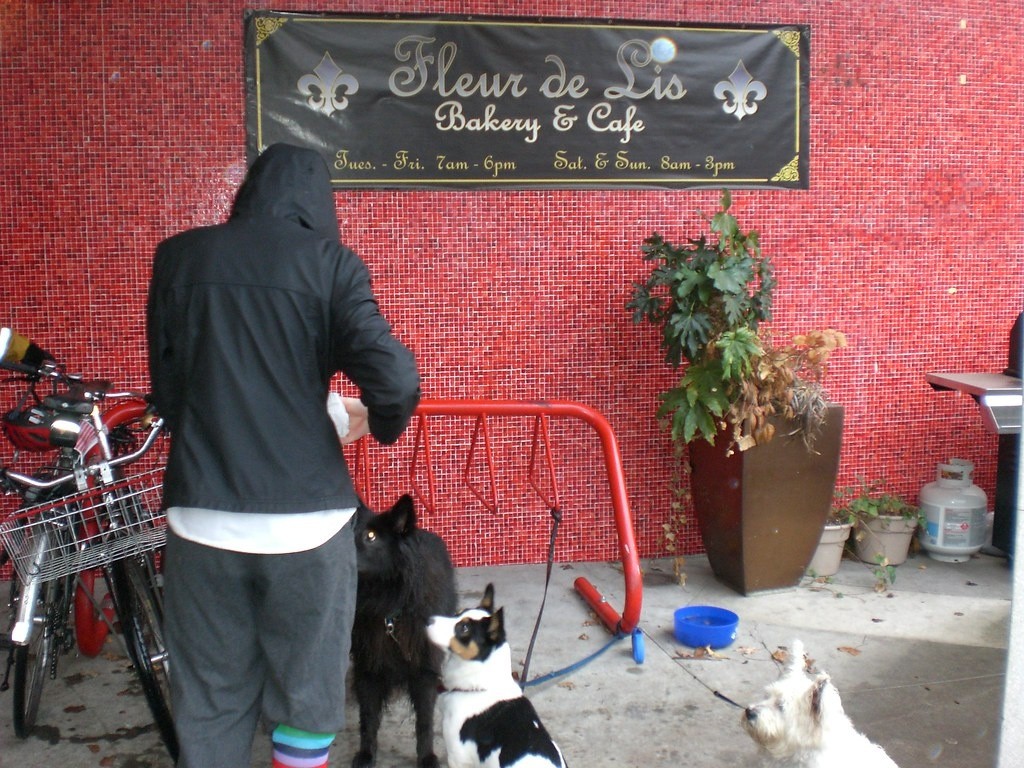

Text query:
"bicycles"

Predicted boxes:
[[1, 325, 180, 763]]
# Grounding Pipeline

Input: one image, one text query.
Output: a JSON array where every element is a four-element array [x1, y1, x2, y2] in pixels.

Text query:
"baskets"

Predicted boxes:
[[0, 466, 169, 589]]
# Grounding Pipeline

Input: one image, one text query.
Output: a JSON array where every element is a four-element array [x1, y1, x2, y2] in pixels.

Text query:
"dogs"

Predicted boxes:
[[741, 637, 898, 767], [346, 495, 569, 768]]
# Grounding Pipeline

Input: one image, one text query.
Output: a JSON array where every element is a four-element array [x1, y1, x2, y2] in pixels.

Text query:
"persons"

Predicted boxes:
[[146, 142, 422, 768]]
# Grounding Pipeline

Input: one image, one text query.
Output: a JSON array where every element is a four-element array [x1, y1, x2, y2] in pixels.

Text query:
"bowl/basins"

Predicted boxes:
[[674, 606, 739, 649]]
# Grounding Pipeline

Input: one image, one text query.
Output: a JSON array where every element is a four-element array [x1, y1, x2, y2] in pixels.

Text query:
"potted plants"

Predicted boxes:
[[629, 185, 932, 596]]
